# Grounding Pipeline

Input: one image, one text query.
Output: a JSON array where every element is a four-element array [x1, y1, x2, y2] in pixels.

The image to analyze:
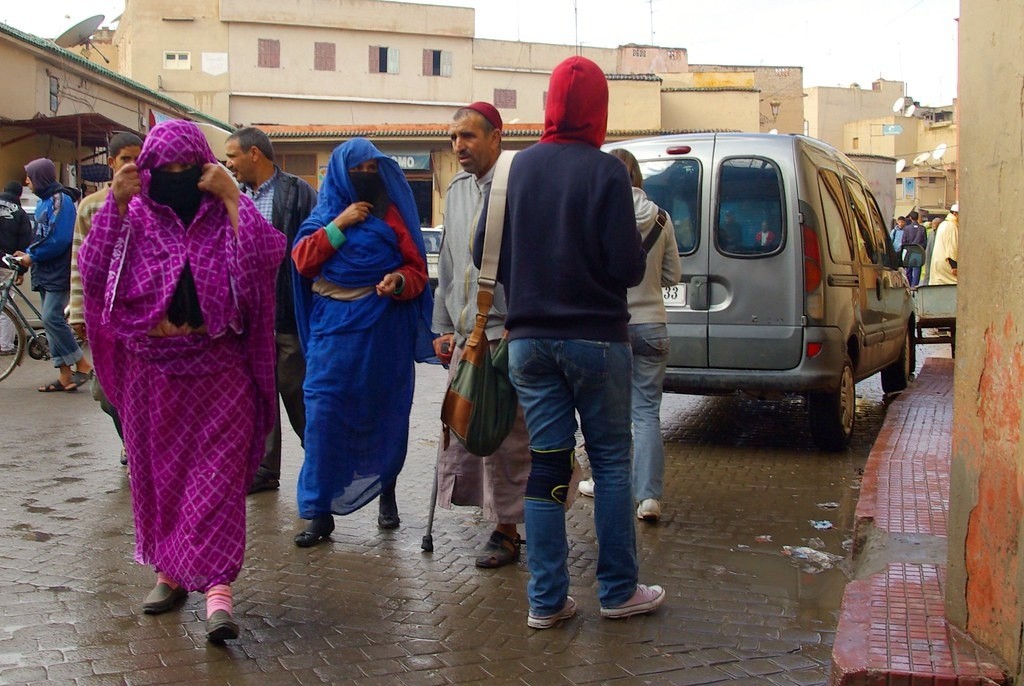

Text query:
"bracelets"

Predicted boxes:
[[392, 272, 404, 295]]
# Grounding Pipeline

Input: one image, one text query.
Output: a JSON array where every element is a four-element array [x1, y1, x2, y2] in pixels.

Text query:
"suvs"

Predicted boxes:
[[599, 127, 925, 454]]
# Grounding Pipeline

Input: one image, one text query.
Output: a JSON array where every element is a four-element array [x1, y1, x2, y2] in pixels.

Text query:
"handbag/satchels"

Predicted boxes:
[[439, 325, 519, 459]]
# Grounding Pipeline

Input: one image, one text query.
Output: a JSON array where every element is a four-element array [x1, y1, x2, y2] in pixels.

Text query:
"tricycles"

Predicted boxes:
[[910, 283, 958, 372]]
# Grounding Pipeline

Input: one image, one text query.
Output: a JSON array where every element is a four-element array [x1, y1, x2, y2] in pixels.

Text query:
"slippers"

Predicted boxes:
[[37, 379, 77, 393], [65, 368, 94, 391]]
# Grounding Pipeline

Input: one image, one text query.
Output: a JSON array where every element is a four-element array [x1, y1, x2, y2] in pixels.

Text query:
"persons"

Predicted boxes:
[[578, 149, 681, 520], [12, 157, 95, 392], [76, 118, 287, 638], [719, 210, 743, 248], [224, 126, 318, 494], [424, 238, 431, 251], [292, 137, 443, 549], [474, 53, 666, 629], [0, 180, 32, 356], [431, 100, 584, 568], [891, 211, 928, 288], [924, 204, 959, 285], [68, 132, 144, 464], [755, 219, 775, 247]]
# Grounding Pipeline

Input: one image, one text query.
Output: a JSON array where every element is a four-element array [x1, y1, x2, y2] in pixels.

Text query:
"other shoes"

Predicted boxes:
[[120, 444, 129, 466], [203, 609, 240, 641], [377, 506, 401, 529], [0, 347, 18, 356], [141, 581, 181, 614], [294, 516, 336, 547], [244, 473, 281, 494]]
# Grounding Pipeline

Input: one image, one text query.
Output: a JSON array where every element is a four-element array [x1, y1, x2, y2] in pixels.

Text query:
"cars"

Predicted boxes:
[[420, 227, 443, 282]]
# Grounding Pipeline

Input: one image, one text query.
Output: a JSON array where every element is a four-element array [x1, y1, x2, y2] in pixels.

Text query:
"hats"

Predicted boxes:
[[950, 202, 959, 213], [453, 102, 503, 132]]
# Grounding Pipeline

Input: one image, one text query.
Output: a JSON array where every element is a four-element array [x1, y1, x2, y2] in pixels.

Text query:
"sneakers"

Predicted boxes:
[[601, 585, 666, 619], [526, 595, 577, 629], [577, 475, 597, 497], [636, 498, 661, 521]]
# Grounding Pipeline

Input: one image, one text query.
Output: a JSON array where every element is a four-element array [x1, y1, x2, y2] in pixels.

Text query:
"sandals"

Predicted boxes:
[[475, 530, 525, 569]]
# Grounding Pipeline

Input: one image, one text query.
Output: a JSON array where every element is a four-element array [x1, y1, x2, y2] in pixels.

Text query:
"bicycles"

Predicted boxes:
[[0, 254, 88, 382]]
